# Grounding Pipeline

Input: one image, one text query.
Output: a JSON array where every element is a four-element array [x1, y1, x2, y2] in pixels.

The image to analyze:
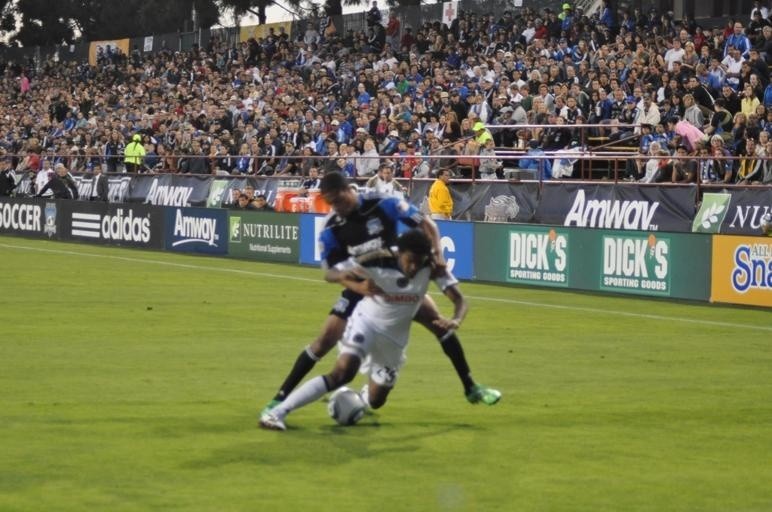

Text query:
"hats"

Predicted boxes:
[[0, 1, 672, 157]]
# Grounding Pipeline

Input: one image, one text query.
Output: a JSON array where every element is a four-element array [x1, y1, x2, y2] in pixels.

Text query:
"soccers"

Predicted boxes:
[[329, 387, 365, 426]]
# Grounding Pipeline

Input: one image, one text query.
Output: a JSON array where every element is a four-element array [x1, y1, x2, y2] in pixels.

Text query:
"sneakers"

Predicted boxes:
[[258, 397, 290, 434], [463, 383, 503, 407], [361, 384, 370, 411]]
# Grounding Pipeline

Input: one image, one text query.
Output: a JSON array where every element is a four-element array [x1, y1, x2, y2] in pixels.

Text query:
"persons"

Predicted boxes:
[[758, 211, 772, 237], [256, 226, 471, 435], [298, 167, 323, 198], [427, 170, 453, 220], [264, 170, 505, 411], [2, 160, 35, 198], [348, 162, 407, 201], [90, 163, 109, 201], [226, 185, 274, 212], [0, 1, 771, 185], [36, 162, 79, 200], [34, 159, 55, 198]]
